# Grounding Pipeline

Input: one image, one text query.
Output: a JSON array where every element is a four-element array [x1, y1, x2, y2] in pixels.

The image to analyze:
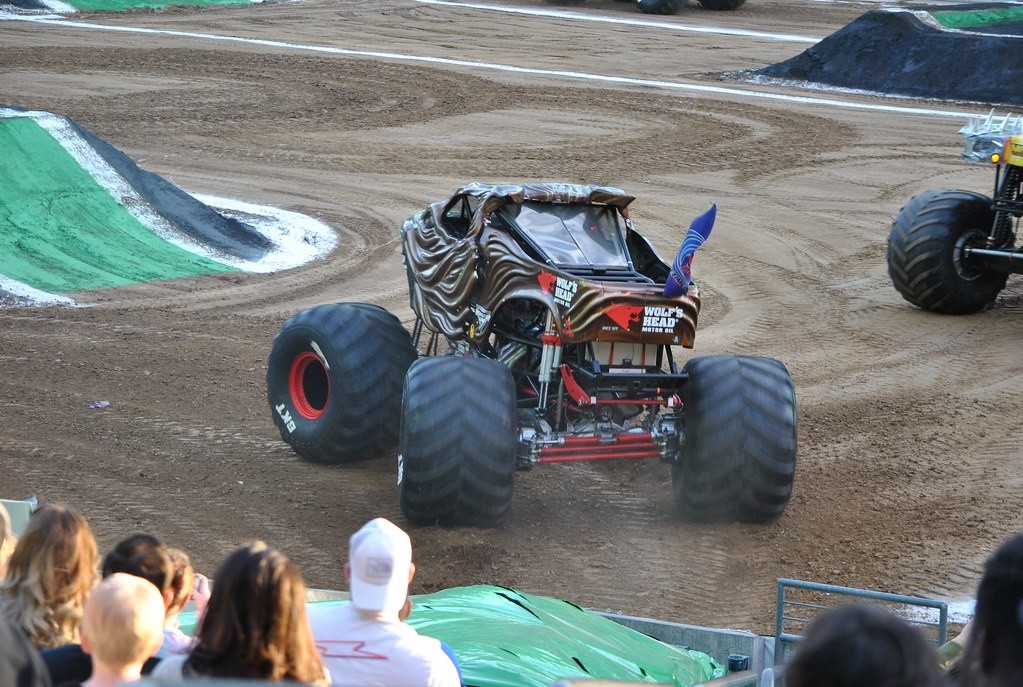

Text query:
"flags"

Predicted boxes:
[[662, 205, 716, 296]]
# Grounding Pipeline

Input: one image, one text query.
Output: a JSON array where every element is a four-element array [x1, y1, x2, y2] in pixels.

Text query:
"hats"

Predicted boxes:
[[349, 517, 412, 610]]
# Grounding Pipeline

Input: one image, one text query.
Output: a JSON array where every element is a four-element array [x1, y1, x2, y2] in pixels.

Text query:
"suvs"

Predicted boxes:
[[263, 179, 799, 526], [885, 134, 1022, 316]]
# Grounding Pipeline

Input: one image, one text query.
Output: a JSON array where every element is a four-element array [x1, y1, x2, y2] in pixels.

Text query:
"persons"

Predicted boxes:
[[0, 501, 330, 687], [787, 603, 948, 687], [936, 534, 1023, 687], [307, 517, 464, 687]]
